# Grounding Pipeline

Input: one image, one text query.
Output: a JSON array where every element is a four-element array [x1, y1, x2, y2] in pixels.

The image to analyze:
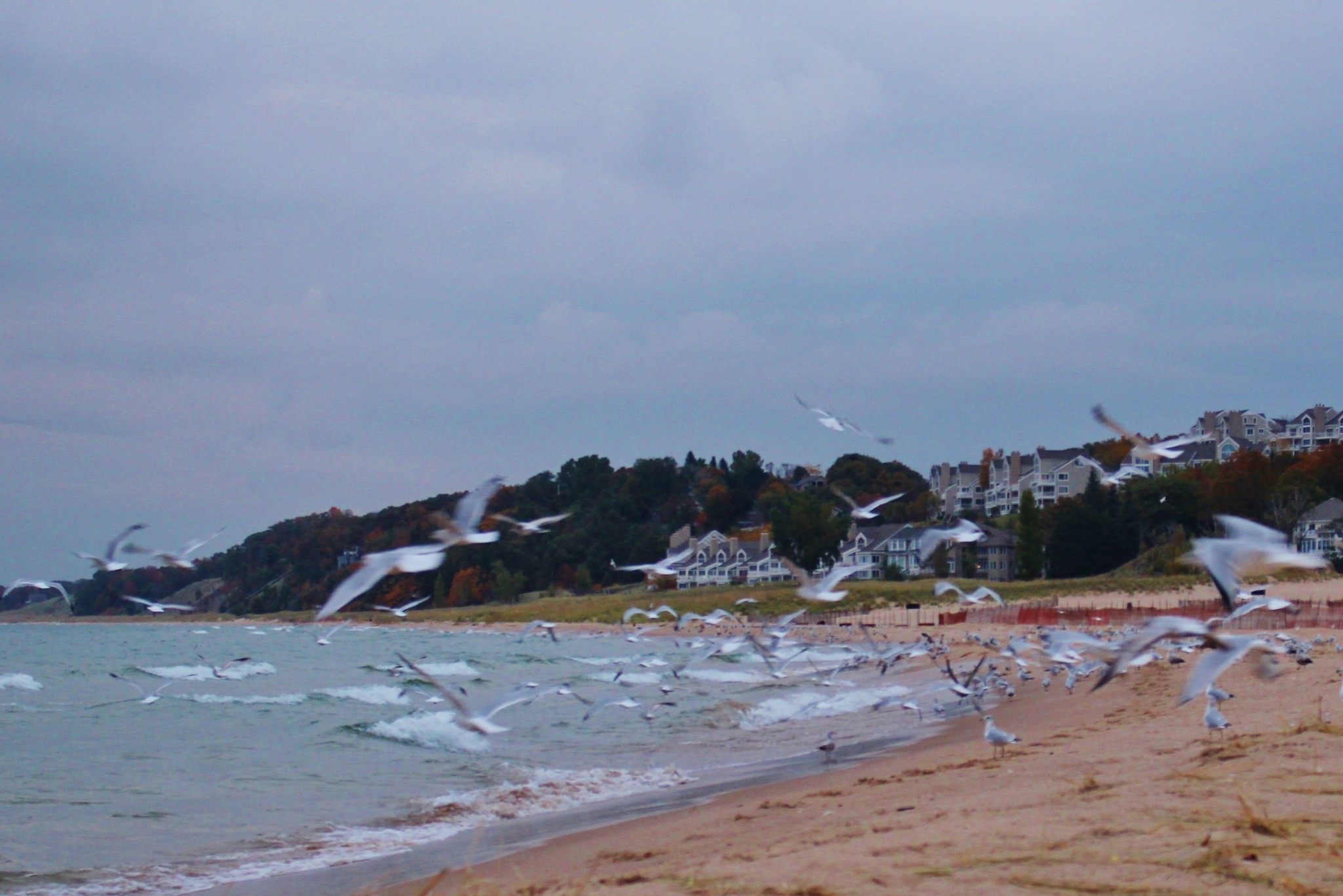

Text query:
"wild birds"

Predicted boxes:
[[1, 469, 591, 738], [818, 731, 838, 763], [793, 393, 894, 445], [1202, 696, 1233, 741], [558, 514, 1342, 731], [1091, 403, 1212, 460], [978, 714, 1023, 757], [1049, 453, 1149, 486], [831, 486, 908, 519]]
